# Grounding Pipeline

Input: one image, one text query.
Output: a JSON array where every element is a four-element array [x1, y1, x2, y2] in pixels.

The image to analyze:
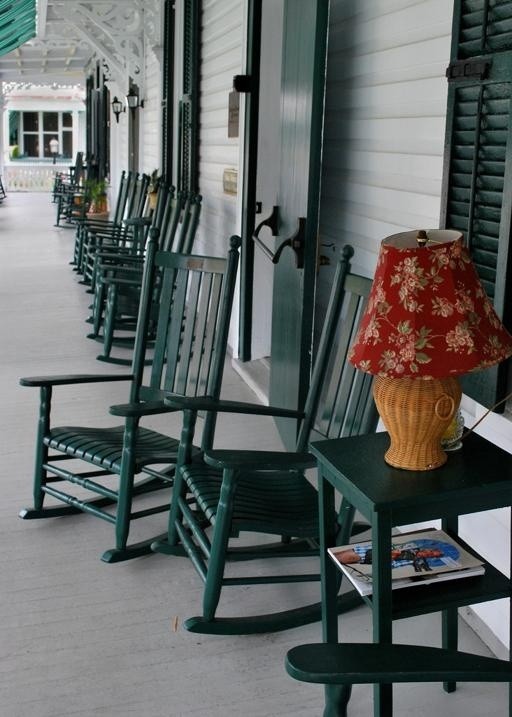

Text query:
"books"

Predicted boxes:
[[326, 528, 487, 598]]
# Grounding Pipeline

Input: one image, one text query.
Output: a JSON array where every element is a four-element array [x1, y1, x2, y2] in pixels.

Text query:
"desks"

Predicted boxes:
[[303, 421, 511, 717]]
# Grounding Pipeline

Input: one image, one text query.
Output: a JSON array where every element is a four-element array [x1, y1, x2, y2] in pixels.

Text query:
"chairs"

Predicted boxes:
[[101, 243, 393, 638], [46, 147, 205, 370], [14, 233, 246, 566]]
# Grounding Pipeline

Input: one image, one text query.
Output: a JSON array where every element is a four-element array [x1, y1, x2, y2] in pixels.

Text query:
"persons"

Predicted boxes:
[[334, 542, 443, 568]]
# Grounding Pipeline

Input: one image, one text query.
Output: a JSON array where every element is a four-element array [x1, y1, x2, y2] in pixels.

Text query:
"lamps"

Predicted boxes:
[[111, 94, 125, 124], [125, 82, 144, 121], [342, 223, 495, 475]]
